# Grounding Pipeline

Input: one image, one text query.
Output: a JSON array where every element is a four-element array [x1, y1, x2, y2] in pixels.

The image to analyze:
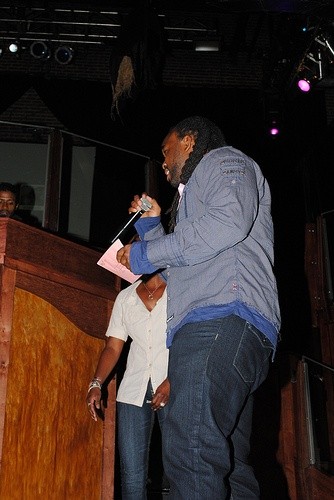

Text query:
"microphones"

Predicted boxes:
[[111, 198, 152, 244]]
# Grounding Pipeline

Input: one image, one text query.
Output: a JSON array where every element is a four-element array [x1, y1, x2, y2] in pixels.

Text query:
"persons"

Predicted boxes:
[[0, 181, 24, 223], [85, 227, 172, 500], [116, 117, 282, 500]]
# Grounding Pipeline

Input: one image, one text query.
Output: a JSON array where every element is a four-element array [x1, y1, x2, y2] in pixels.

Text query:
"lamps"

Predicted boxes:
[[297, 76, 311, 92], [9, 39, 80, 65]]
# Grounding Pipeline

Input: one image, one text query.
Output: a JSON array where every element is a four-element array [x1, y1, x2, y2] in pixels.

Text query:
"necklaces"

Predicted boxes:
[[142, 279, 164, 300]]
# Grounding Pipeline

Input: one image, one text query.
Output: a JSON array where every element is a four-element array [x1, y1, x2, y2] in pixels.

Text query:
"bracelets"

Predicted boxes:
[[87, 376, 105, 393]]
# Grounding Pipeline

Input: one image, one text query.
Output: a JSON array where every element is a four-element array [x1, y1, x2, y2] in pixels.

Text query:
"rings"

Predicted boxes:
[[159, 402, 166, 407]]
